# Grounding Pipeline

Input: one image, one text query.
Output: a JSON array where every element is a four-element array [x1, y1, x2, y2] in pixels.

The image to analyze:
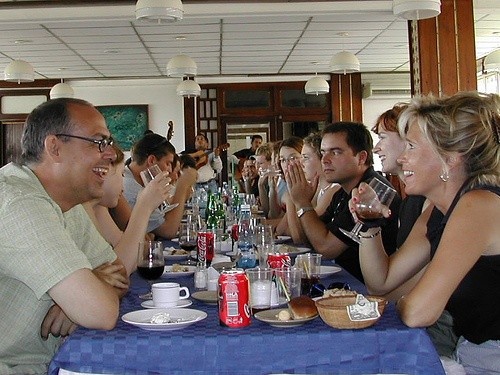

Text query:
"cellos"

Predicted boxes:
[[165, 120, 175, 141]]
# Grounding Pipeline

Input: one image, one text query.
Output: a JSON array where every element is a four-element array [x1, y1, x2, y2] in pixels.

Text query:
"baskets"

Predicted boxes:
[[315, 295, 388, 329]]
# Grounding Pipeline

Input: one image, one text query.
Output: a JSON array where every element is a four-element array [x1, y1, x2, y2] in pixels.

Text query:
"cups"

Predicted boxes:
[[151, 281, 190, 305], [249, 217, 324, 309]]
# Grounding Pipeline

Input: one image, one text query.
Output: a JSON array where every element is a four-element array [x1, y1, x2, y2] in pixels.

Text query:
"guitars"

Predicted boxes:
[[233, 158, 249, 183], [186, 142, 230, 171]]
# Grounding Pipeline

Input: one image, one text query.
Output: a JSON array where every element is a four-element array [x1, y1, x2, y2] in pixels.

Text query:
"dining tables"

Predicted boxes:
[[47, 205, 446, 375]]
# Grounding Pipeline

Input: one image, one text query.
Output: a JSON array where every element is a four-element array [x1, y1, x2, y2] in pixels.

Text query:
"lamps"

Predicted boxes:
[[50, 68, 75, 99], [166, 36, 197, 77], [305, 62, 329, 96], [328, 33, 360, 75], [134, 0, 184, 24], [176, 77, 201, 99], [393, 0, 441, 20], [5, 40, 34, 84]]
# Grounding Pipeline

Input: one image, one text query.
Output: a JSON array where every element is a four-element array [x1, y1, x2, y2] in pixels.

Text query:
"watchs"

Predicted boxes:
[[296, 206, 313, 218]]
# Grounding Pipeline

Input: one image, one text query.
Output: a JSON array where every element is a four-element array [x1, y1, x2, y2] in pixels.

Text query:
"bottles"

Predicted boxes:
[[236, 205, 256, 267], [199, 181, 245, 230]]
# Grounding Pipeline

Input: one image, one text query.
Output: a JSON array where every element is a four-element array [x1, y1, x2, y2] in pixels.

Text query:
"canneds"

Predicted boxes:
[[267, 253, 292, 297], [218, 271, 251, 328], [233, 224, 246, 242], [197, 231, 215, 262]]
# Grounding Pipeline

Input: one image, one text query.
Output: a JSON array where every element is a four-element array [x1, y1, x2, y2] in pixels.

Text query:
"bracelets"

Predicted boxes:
[[358, 229, 382, 239], [244, 179, 251, 182]]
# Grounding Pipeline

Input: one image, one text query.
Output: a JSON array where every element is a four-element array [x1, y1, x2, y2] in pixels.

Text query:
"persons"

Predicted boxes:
[[0, 97, 129, 375], [231, 134, 341, 244], [373, 103, 458, 357], [82, 147, 172, 276], [121, 135, 225, 240], [348, 92, 500, 375], [285, 121, 401, 284]]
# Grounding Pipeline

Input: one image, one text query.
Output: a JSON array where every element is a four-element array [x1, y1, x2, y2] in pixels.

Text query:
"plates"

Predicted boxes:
[[162, 263, 198, 278], [292, 261, 343, 276], [122, 307, 208, 332], [253, 307, 320, 328], [161, 248, 191, 260], [310, 294, 321, 302], [214, 240, 245, 254], [140, 299, 193, 309], [274, 233, 291, 242], [266, 242, 310, 256], [192, 289, 218, 305]]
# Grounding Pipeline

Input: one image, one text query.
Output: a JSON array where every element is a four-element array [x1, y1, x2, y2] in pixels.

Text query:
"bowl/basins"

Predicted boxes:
[[315, 296, 388, 330]]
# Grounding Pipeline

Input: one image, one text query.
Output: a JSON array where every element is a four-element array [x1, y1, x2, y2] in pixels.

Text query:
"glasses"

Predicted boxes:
[[55, 134, 114, 153], [280, 156, 300, 163]]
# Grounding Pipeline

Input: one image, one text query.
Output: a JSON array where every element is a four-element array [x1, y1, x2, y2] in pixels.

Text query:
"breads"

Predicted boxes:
[[287, 297, 318, 319]]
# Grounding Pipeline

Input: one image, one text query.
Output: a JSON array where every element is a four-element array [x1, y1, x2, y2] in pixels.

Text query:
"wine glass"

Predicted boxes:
[[259, 161, 284, 180], [140, 165, 180, 214], [176, 169, 199, 268], [136, 241, 165, 299], [339, 178, 398, 245]]
